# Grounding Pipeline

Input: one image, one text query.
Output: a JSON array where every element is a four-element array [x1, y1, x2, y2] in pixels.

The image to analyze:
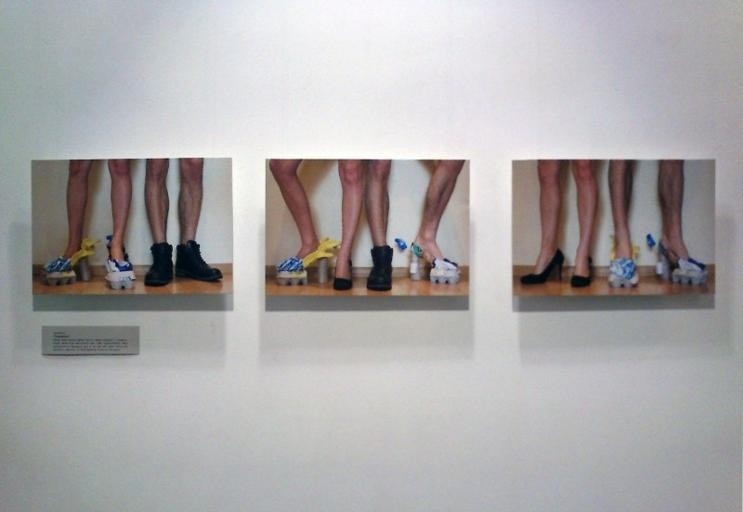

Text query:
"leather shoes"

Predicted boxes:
[[144, 242, 174, 287], [174, 243, 222, 281]]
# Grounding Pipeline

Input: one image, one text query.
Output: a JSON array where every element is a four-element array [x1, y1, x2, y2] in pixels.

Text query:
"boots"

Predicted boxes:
[[366, 245, 395, 290]]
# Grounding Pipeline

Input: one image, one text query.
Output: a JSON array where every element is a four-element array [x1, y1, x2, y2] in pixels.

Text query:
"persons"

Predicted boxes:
[[268, 159, 338, 284], [331, 160, 362, 290], [408, 161, 466, 284], [41, 160, 133, 275], [607, 161, 706, 282], [517, 160, 596, 288], [363, 160, 456, 290], [143, 158, 223, 285]]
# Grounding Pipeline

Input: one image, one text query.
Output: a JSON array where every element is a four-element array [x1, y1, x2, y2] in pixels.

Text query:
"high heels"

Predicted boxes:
[[409, 239, 461, 285], [103, 236, 137, 291], [570, 254, 594, 288], [654, 240, 709, 286], [42, 238, 97, 285], [521, 248, 566, 285], [606, 239, 642, 289], [275, 240, 336, 287], [332, 255, 354, 289]]
